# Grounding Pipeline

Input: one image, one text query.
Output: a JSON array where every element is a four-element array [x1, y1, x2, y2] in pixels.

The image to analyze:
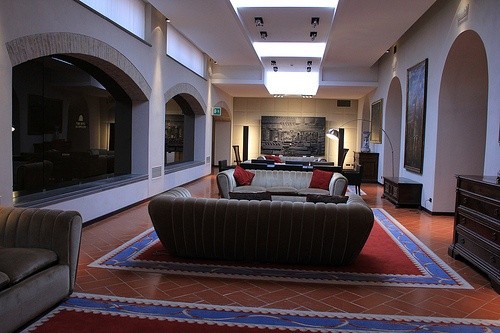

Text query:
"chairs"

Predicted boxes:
[[342, 164, 362, 195], [232, 145, 241, 165]]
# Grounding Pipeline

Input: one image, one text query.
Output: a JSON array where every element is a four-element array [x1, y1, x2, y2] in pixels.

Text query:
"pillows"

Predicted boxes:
[[308, 168, 334, 191], [233, 165, 251, 185], [246, 171, 256, 185]]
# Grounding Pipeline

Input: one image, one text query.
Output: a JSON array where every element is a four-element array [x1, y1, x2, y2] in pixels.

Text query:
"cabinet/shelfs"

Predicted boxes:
[[381, 176, 423, 209], [353, 151, 379, 183], [448, 175, 500, 293]]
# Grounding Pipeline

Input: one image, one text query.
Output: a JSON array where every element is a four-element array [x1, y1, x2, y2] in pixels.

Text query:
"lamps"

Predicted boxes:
[[326, 118, 395, 176]]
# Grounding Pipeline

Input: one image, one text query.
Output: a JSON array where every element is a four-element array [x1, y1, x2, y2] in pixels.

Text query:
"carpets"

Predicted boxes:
[[20, 291, 500, 333], [346, 184, 367, 195], [87, 208, 474, 290]]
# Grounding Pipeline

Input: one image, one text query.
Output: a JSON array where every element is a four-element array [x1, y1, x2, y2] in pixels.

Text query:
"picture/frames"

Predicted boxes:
[[370, 98, 383, 144], [402, 57, 427, 175]]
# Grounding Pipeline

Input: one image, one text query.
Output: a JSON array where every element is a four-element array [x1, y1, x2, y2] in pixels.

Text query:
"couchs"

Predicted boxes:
[[0, 204, 83, 333], [148, 169, 374, 266], [13, 139, 115, 193]]
